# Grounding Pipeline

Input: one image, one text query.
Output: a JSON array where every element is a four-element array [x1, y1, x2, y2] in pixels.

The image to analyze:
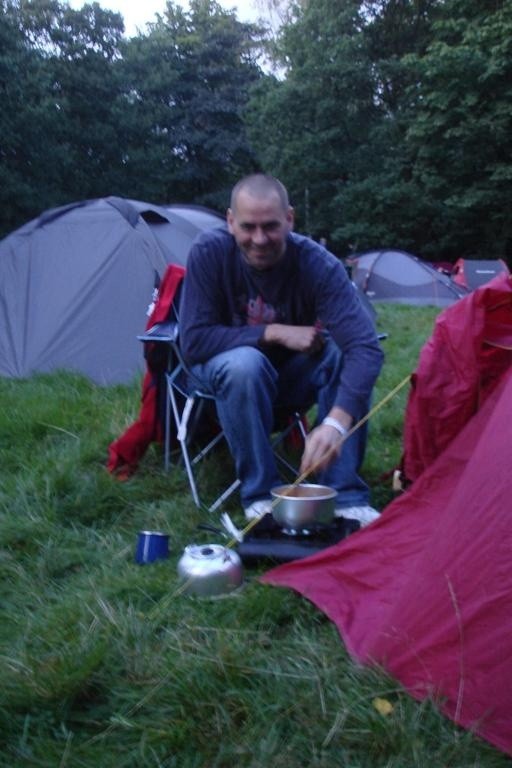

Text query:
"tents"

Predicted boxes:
[[1, 196, 230, 388], [256, 269, 511, 757], [451, 257, 510, 291], [341, 248, 469, 306]]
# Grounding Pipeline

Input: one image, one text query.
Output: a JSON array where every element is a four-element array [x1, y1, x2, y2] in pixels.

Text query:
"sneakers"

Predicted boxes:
[[331, 501, 378, 528], [244, 498, 274, 523]]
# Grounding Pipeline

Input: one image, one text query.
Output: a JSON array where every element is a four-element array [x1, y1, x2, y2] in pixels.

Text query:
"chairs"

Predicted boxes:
[[137, 268, 314, 509]]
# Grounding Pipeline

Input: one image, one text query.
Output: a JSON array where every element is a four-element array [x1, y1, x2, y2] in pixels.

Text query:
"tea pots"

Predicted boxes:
[[176, 523, 247, 601]]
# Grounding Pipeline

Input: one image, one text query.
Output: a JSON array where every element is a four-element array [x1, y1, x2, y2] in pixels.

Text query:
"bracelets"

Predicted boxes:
[[321, 417, 348, 435]]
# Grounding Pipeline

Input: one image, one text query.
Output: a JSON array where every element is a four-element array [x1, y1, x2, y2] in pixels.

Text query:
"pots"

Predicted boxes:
[[270, 483, 338, 529]]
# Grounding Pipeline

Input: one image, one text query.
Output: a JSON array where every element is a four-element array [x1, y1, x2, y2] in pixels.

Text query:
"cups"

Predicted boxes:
[[134, 530, 170, 565]]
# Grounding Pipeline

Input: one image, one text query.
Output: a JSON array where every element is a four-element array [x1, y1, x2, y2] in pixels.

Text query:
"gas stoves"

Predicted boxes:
[[240, 512, 360, 568]]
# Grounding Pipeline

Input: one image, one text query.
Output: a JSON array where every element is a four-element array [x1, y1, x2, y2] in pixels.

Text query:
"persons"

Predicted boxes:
[[175, 175, 381, 529]]
